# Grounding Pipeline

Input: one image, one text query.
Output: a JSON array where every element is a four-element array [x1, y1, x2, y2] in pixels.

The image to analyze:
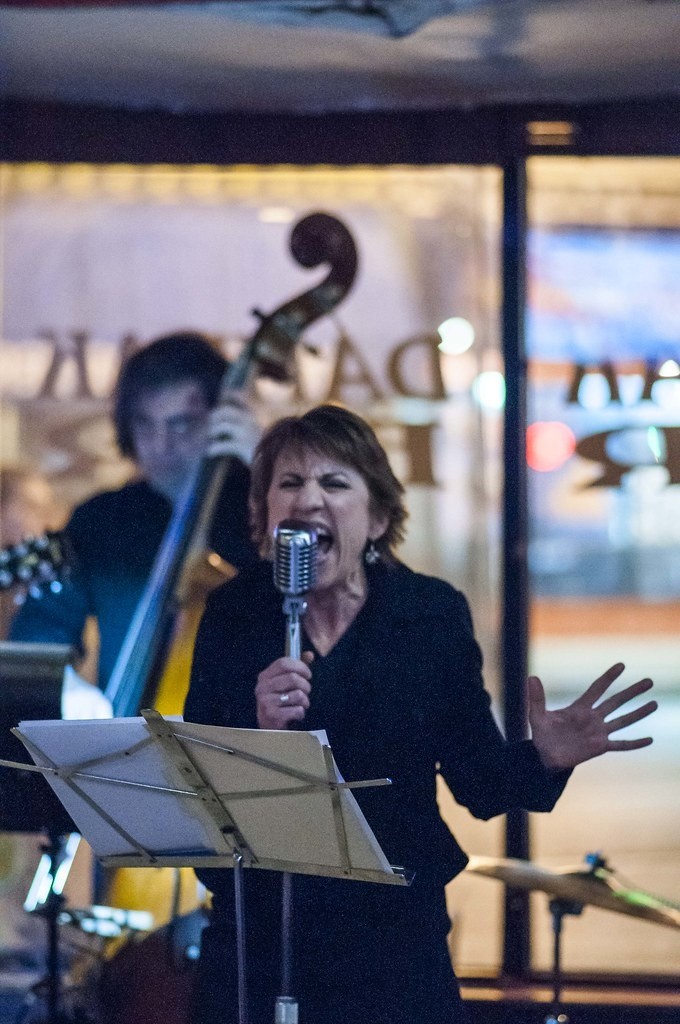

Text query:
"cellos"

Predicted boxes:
[[90, 210, 358, 972]]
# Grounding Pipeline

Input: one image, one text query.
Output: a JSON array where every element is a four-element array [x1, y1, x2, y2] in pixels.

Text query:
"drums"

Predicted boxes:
[[75, 905, 212, 1024]]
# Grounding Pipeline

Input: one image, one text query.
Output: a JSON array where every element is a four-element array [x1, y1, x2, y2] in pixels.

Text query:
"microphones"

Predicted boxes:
[[273, 518, 319, 662]]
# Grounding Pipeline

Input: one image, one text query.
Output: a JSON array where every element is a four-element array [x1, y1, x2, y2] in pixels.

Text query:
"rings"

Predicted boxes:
[[279, 693, 289, 708]]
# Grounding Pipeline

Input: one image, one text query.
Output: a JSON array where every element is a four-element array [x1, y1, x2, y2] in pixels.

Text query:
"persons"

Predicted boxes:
[[59, 330, 265, 693], [183, 405, 660, 1024]]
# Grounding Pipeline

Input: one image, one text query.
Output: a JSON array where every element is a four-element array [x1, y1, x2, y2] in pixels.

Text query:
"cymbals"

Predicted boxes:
[[464, 854, 680, 931]]
